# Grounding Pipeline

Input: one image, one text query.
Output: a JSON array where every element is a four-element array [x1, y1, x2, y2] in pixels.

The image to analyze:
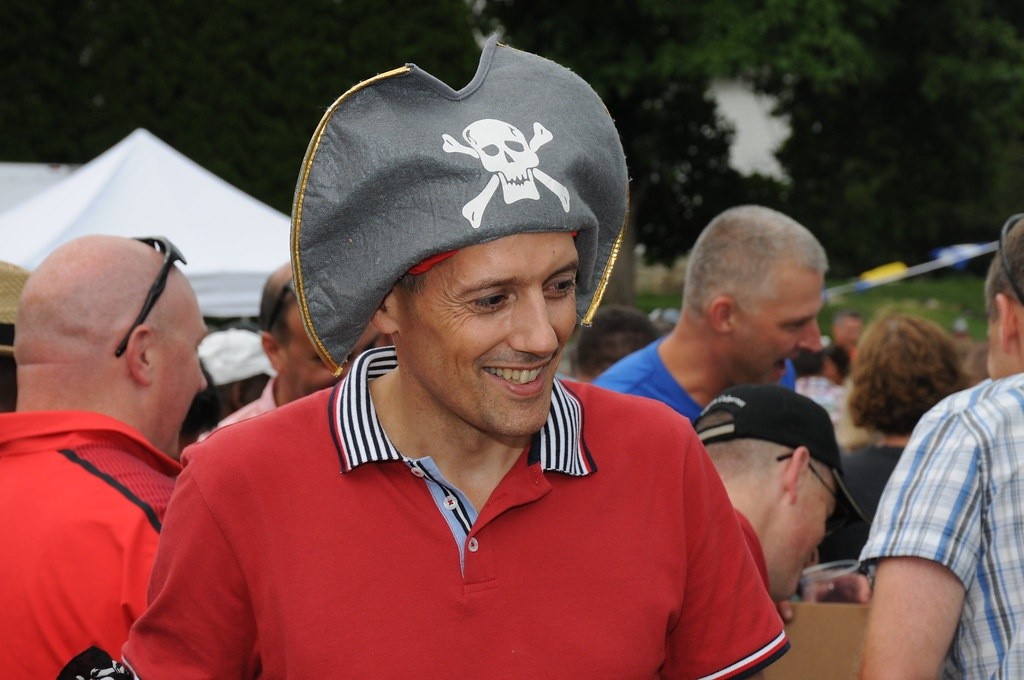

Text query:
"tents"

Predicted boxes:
[[1, 129, 292, 323]]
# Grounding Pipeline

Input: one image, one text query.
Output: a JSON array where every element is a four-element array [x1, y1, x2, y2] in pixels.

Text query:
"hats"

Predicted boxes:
[[692, 383, 873, 525], [825, 345, 850, 376], [289, 34, 630, 375]]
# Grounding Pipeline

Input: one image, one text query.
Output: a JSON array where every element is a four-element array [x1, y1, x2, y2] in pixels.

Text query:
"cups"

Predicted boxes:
[[799, 559, 861, 603]]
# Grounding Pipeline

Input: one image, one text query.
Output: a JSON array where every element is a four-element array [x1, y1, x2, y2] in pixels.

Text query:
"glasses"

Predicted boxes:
[[999, 213, 1024, 305], [115, 235, 186, 358], [777, 452, 849, 534]]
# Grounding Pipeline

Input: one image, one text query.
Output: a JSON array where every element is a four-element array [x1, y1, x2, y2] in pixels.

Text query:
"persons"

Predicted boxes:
[[115, 44, 789, 680], [0, 207, 1023, 680]]
